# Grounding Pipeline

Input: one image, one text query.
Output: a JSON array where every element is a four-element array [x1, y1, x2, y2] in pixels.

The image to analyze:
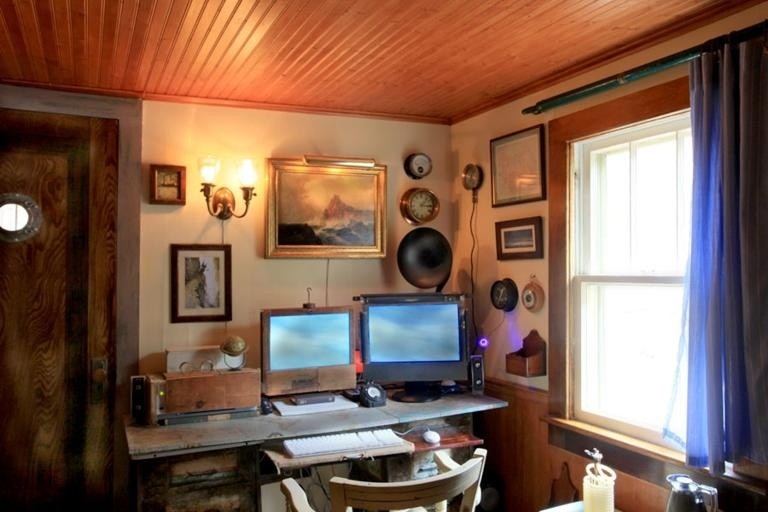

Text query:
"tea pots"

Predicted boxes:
[[665, 474, 718, 512]]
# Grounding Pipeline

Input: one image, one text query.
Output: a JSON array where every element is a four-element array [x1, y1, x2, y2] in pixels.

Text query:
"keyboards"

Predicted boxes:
[[283, 428, 405, 459]]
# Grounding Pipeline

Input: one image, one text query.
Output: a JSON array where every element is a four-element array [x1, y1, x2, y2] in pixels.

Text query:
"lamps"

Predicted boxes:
[[197, 150, 259, 219]]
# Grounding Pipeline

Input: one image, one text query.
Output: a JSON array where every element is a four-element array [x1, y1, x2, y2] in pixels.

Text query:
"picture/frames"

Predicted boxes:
[[265, 157, 389, 261], [170, 243, 233, 324], [489, 122, 545, 208], [495, 216, 542, 262], [149, 163, 187, 205]]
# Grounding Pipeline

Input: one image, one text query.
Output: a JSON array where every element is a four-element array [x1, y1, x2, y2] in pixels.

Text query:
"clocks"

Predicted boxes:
[[490, 278, 518, 312], [400, 187, 441, 224], [461, 164, 482, 190]]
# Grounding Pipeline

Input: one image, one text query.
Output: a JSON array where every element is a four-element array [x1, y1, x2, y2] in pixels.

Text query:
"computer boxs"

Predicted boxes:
[[385, 448, 450, 512]]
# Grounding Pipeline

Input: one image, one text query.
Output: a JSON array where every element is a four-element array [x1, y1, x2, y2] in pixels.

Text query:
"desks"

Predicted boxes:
[[119, 377, 509, 512], [539, 498, 628, 512]]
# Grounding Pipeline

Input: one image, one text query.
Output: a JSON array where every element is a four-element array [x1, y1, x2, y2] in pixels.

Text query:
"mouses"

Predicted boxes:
[[422, 430, 441, 443]]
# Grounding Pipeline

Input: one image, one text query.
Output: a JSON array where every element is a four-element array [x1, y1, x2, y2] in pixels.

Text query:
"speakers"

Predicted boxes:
[[471, 354, 484, 395], [129, 374, 147, 427]]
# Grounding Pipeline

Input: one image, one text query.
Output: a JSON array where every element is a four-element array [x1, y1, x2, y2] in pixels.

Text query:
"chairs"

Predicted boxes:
[[280, 448, 489, 512]]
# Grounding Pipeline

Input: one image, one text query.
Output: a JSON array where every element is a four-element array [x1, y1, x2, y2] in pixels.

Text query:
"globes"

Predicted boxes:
[[223, 335, 247, 352]]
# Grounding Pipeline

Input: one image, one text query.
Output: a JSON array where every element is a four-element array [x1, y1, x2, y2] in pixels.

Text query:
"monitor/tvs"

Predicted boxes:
[[360, 293, 469, 403], [259, 305, 356, 406]]
